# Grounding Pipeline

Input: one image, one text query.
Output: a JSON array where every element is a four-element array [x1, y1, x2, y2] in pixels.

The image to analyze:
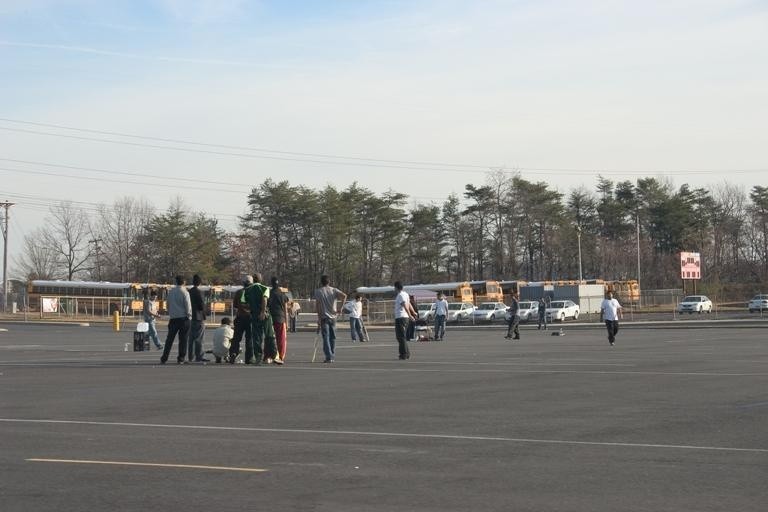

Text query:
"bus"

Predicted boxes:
[[24, 277, 294, 318], [349, 277, 644, 309]]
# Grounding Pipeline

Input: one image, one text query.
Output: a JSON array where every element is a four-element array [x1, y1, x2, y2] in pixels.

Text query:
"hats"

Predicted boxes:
[[271, 277, 279, 285], [242, 275, 253, 285], [191, 274, 202, 283], [253, 272, 262, 282]]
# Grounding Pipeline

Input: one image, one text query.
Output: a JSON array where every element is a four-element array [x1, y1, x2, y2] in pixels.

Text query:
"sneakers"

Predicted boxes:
[[157, 344, 165, 350], [350, 338, 368, 343], [434, 337, 444, 342], [609, 342, 615, 346], [323, 356, 336, 364], [158, 356, 290, 369], [396, 353, 411, 362], [536, 326, 547, 331], [503, 335, 521, 341]]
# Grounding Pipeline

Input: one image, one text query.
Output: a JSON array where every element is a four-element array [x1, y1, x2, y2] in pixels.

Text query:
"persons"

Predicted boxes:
[[144, 291, 164, 349], [288, 302, 297, 332], [504, 295, 520, 339], [316, 276, 347, 363], [600, 291, 623, 345], [344, 296, 366, 343], [537, 298, 547, 330], [406, 296, 418, 341], [433, 293, 449, 341], [394, 282, 418, 360], [161, 273, 289, 365]]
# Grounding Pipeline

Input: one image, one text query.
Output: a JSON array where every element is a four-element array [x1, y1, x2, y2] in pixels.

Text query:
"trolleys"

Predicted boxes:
[[411, 320, 438, 344]]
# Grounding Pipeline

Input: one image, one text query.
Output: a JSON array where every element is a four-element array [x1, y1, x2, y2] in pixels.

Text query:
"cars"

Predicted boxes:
[[674, 295, 715, 315], [574, 224, 585, 283], [748, 294, 767, 313], [416, 299, 582, 324]]
